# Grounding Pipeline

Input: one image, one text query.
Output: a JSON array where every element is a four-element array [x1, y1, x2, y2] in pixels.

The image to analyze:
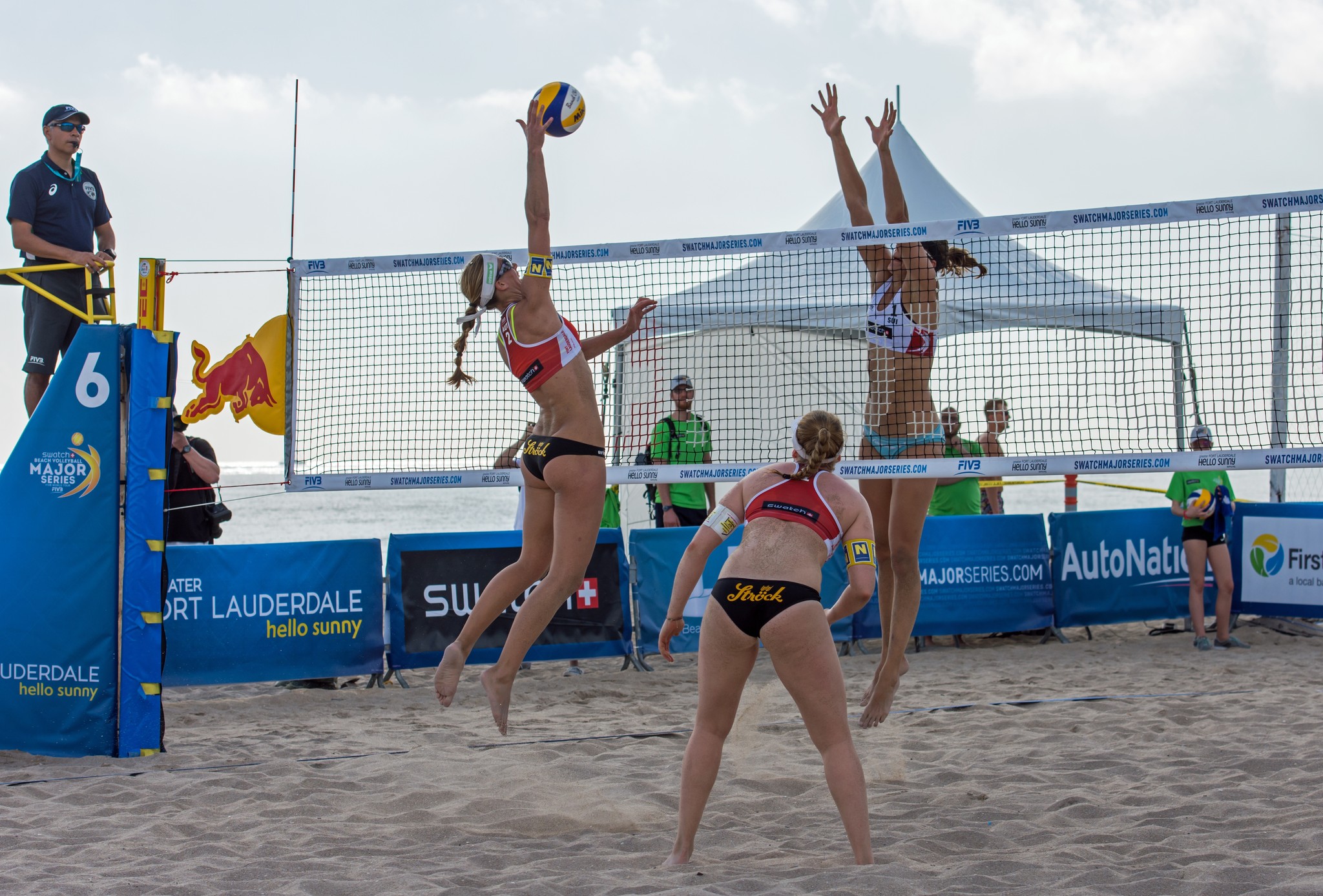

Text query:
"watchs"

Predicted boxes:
[[103, 248, 117, 260], [663, 505, 672, 512], [180, 444, 191, 455]]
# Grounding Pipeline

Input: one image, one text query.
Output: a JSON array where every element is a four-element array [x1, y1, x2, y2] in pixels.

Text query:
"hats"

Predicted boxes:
[[1190, 426, 1212, 444], [42, 104, 90, 127], [670, 375, 693, 390]]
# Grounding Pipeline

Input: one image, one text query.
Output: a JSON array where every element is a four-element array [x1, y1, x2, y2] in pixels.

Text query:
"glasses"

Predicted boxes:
[[1003, 412, 1009, 416], [496, 257, 513, 282], [1193, 441, 1210, 448], [674, 387, 691, 394], [47, 122, 85, 134]]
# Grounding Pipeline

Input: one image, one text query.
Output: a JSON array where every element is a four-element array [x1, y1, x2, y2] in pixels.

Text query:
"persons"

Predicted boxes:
[[922, 407, 985, 648], [651, 374, 715, 528], [974, 398, 1011, 515], [1165, 425, 1251, 651], [658, 410, 876, 866], [494, 423, 584, 676], [434, 99, 657, 736], [810, 81, 987, 729], [6, 104, 116, 421], [168, 404, 220, 545]]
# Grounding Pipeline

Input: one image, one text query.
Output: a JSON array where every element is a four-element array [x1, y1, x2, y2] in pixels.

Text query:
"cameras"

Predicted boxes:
[[172, 415, 188, 432]]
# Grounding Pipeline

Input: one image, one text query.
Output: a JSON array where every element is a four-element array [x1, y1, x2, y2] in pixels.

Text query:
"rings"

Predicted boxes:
[[85, 263, 89, 268]]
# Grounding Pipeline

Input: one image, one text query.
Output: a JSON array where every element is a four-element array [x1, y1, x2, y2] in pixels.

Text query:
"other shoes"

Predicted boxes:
[[1193, 635, 1215, 651], [1214, 636, 1251, 651]]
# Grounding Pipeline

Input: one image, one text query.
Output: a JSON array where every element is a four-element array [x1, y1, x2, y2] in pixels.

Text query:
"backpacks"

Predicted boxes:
[[642, 415, 708, 503]]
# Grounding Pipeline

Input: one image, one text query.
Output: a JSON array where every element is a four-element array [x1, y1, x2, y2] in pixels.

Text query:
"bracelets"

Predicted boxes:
[[1184, 510, 1191, 519], [665, 615, 688, 630]]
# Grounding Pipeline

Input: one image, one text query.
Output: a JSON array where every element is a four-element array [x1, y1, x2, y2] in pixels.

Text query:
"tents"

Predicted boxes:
[[610, 123, 1187, 510]]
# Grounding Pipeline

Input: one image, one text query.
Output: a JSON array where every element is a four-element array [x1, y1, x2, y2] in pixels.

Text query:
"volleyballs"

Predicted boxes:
[[532, 81, 586, 138], [1186, 488, 1217, 519]]
